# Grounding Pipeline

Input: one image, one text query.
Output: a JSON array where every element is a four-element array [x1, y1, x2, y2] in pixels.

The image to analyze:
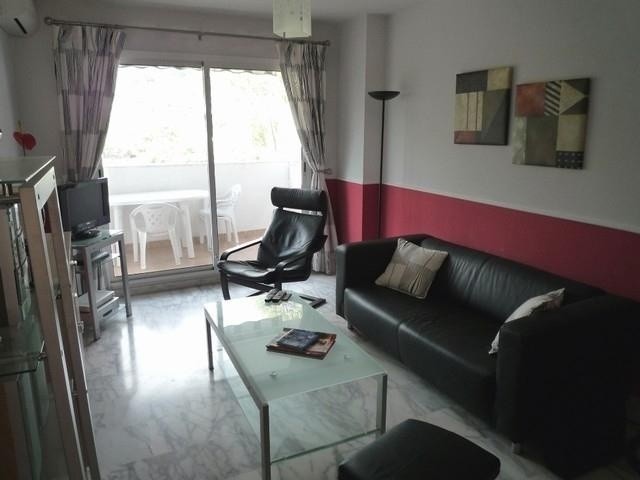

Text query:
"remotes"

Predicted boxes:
[[264, 288, 279, 302], [281, 293, 292, 301], [271, 290, 287, 303]]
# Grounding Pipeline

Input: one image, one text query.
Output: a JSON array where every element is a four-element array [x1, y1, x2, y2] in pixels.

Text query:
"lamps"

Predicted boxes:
[[368, 90, 400, 239], [272, 1, 311, 40]]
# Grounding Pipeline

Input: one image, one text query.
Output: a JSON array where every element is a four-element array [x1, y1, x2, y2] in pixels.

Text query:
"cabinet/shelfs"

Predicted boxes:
[[0, 154, 100, 479], [70, 228, 132, 341]]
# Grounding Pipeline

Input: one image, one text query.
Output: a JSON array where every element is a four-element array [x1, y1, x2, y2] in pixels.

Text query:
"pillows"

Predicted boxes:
[[374, 238, 449, 300], [488, 287, 566, 355]]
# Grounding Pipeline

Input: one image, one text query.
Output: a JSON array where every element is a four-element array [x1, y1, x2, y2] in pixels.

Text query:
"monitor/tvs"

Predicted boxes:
[[50, 178, 111, 240]]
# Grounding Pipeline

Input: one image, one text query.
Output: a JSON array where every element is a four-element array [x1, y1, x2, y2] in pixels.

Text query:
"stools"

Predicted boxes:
[[337, 418, 501, 480]]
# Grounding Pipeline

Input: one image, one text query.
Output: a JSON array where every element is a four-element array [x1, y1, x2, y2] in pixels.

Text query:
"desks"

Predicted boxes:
[[108, 189, 212, 258]]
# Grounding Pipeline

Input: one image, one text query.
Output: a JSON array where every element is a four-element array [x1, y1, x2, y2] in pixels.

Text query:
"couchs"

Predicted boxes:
[[333, 233, 639, 479]]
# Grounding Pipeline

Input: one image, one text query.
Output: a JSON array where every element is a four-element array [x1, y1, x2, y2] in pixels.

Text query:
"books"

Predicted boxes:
[[278, 330, 319, 351]]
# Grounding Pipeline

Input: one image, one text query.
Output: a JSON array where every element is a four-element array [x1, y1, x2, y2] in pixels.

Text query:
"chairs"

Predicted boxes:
[[128, 203, 182, 269], [216, 186, 328, 308], [198, 183, 239, 252]]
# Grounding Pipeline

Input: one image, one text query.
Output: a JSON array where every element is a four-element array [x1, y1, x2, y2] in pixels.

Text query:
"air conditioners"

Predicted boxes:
[[0, 1, 38, 36]]
